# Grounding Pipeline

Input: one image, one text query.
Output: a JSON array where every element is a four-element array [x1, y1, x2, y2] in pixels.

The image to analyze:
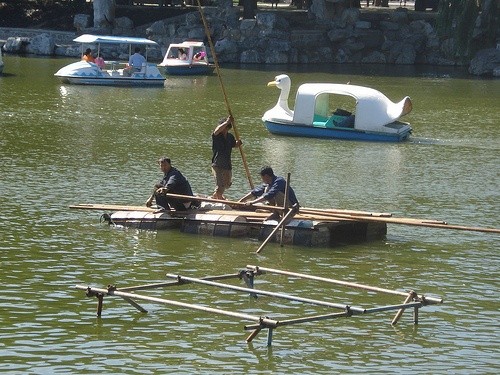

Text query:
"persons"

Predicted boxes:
[[93, 53, 105, 70], [237, 165, 299, 224], [129, 48, 146, 72], [81, 47, 95, 63], [178, 49, 187, 59], [145, 158, 194, 212], [210, 114, 244, 200]]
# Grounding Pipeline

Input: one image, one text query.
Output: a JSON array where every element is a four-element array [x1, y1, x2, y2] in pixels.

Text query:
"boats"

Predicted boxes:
[[100, 200, 388, 247], [157, 41, 216, 75], [52, 33, 167, 86], [262, 73, 413, 143]]
